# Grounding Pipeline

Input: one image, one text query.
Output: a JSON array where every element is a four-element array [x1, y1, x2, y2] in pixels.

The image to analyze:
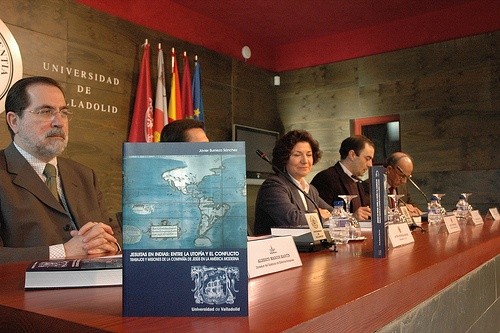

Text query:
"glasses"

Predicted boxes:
[[393, 167, 412, 182], [13, 106, 73, 122]]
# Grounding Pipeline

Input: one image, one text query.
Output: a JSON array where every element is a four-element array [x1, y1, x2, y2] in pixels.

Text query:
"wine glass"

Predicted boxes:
[[462, 193, 474, 217], [338, 194, 361, 240], [433, 194, 447, 223], [387, 194, 408, 224]]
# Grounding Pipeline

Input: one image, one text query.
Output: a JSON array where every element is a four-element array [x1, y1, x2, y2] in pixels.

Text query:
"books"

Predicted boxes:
[[270, 225, 330, 237], [24, 258, 123, 290]]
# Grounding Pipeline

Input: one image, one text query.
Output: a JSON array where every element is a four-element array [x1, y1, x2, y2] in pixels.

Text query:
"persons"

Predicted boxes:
[[364, 152, 424, 216], [253, 130, 340, 235], [310, 134, 375, 222], [160, 119, 211, 142], [0, 76, 123, 263]]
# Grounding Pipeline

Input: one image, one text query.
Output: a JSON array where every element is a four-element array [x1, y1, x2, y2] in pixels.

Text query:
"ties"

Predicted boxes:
[[43, 163, 66, 212]]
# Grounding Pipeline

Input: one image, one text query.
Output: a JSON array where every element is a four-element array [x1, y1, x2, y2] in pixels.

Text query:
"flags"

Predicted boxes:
[[154, 43, 169, 143], [168, 47, 206, 134], [128, 38, 155, 142]]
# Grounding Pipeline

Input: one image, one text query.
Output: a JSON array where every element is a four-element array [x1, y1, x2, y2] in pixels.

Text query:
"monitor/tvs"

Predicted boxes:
[[232, 124, 279, 179]]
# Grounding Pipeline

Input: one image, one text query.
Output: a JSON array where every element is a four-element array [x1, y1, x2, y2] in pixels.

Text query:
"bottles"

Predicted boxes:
[[455, 195, 468, 220], [428, 196, 442, 225], [330, 201, 350, 245]]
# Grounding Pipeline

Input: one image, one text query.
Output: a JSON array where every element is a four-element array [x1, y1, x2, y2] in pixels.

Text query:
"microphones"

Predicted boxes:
[[397, 166, 430, 221], [255, 148, 338, 252]]
